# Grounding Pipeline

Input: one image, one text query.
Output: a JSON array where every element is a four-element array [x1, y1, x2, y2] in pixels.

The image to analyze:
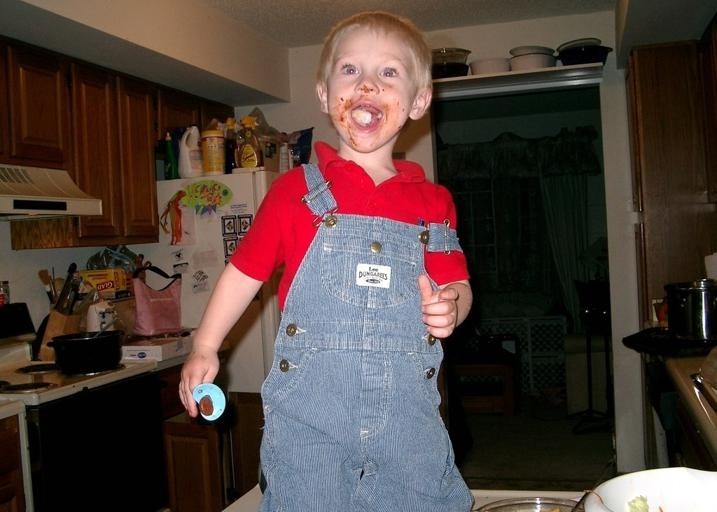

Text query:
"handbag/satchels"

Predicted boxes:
[[131, 266, 182, 337]]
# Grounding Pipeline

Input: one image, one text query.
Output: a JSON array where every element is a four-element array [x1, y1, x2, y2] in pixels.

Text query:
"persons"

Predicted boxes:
[[177, 11, 476, 512]]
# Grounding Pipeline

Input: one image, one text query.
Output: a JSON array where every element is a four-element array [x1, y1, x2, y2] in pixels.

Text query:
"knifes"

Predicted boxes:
[[50, 261, 95, 313]]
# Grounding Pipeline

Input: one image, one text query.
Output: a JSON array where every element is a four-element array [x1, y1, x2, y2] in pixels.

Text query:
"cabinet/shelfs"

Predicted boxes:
[[0, 36, 234, 245], [0, 336, 231, 512], [624, 42, 712, 469]]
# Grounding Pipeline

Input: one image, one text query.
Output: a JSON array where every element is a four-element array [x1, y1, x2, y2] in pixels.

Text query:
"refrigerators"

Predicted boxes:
[[153, 172, 295, 393]]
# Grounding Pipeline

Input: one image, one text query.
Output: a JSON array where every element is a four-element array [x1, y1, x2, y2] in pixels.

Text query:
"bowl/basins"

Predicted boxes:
[[428, 36, 614, 82], [475, 497, 581, 512]]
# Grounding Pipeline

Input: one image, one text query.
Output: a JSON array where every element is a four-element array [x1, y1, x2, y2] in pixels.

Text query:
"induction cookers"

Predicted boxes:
[[0, 343, 158, 407]]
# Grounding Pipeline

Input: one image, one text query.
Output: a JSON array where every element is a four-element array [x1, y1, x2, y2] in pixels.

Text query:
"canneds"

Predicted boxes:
[[0, 281, 10, 305]]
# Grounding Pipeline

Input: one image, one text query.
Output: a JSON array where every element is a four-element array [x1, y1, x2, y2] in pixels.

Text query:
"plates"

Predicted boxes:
[[584, 467, 717, 512]]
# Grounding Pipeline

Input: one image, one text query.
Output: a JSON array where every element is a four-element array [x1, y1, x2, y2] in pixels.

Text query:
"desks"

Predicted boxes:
[[222, 482, 588, 511], [453, 363, 515, 416]]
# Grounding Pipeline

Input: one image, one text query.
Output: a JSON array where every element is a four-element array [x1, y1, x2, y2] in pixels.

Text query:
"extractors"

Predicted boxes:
[[0, 164, 103, 219]]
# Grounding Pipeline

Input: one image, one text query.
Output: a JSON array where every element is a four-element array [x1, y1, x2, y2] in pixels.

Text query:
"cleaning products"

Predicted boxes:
[[177, 125, 202, 177], [224, 117, 235, 168], [234, 115, 261, 167]]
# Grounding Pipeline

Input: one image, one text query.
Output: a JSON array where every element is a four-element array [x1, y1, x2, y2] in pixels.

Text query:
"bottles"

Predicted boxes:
[[277, 138, 291, 174], [202, 131, 225, 175]]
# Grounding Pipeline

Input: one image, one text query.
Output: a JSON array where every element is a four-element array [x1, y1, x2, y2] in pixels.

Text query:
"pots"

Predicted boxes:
[[665, 281, 716, 342], [48, 330, 127, 375]]
